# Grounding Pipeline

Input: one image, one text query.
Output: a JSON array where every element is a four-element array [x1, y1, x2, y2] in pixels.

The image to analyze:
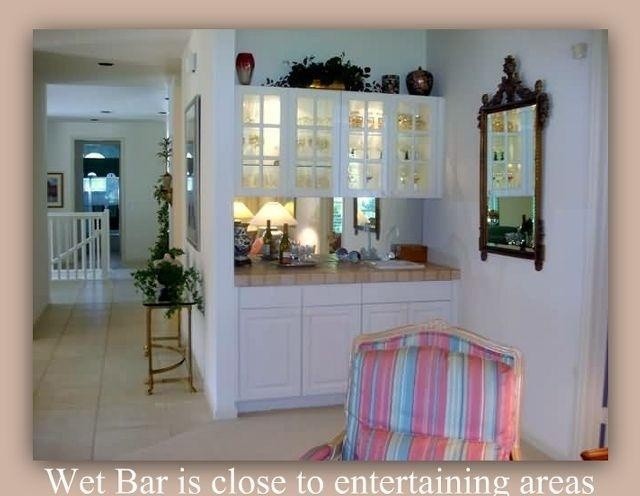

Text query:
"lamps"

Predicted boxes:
[[233, 202, 253, 258], [247, 203, 298, 259]]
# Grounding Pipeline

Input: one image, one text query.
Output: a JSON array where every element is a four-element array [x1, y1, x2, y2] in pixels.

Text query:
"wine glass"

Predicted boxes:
[[289, 241, 316, 264]]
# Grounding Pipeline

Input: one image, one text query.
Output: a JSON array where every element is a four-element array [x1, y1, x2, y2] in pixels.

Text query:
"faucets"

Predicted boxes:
[[364, 222, 371, 251], [374, 225, 399, 260]]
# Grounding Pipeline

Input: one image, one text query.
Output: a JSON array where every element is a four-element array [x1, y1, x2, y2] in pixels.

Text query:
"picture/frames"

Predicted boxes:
[[46, 173, 64, 208]]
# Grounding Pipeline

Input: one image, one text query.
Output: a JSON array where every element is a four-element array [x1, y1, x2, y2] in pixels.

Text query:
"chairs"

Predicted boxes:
[[297, 316, 525, 463]]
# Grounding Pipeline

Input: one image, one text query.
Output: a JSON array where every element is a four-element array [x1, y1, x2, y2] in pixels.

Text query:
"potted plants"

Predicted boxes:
[[131, 249, 207, 318]]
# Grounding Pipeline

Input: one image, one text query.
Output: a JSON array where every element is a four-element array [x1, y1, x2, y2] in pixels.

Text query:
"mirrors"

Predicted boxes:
[[233, 199, 425, 264], [352, 198, 382, 240], [476, 53, 550, 272]]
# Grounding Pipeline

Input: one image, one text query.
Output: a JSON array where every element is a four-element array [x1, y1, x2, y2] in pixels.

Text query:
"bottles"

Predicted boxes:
[[262, 220, 276, 260], [279, 223, 292, 264]]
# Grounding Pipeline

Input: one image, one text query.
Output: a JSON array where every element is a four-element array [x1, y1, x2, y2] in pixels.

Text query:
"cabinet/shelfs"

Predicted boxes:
[[238, 285, 463, 406], [484, 111, 535, 200], [235, 86, 443, 201]]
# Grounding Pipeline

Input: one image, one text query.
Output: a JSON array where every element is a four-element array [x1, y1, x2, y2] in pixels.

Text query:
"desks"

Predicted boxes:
[[140, 297, 198, 397]]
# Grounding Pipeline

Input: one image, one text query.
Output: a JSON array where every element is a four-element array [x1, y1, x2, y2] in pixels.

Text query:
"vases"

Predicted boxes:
[[236, 51, 255, 84]]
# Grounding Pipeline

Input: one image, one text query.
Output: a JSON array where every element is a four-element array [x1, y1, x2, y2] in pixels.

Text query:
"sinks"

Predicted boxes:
[[369, 259, 426, 269]]
